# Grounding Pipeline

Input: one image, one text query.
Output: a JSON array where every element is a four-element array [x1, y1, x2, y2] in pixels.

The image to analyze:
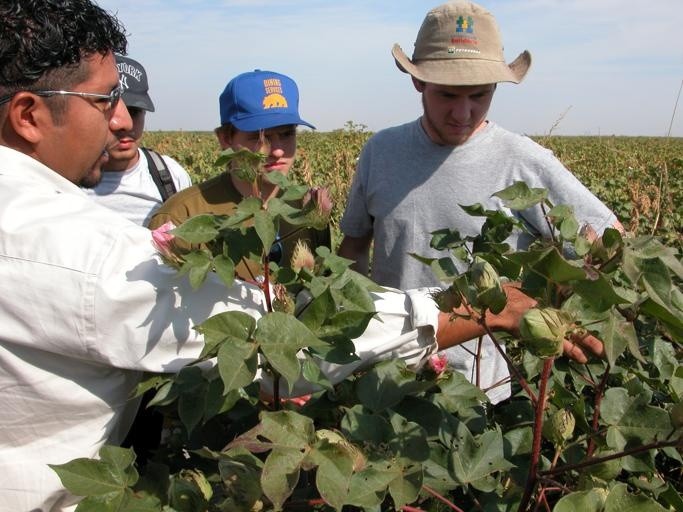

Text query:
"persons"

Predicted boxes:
[[0, 0, 605, 512], [326, 1, 628, 436], [80, 55, 192, 228], [148, 66, 332, 292]]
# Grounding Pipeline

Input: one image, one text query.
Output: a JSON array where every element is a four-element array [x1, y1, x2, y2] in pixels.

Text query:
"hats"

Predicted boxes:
[[390, 0, 533, 89], [219, 69, 317, 135], [115, 55, 156, 114]]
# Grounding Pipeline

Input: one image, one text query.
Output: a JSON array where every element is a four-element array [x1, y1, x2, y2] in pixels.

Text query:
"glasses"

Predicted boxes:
[[0, 78, 121, 110]]
